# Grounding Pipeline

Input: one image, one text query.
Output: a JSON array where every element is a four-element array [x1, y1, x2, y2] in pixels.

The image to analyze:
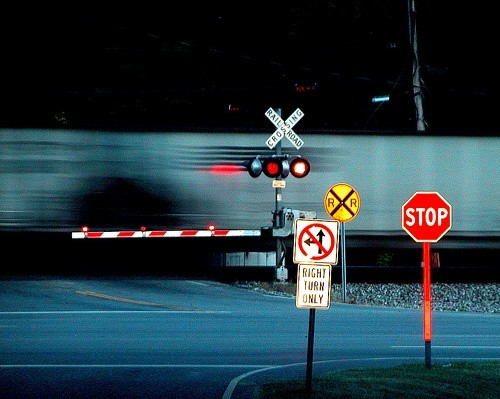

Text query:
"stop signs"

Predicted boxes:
[[402, 192, 453, 243]]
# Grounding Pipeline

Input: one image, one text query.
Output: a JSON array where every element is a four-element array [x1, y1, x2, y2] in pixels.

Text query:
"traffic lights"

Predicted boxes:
[[247, 153, 310, 180]]
